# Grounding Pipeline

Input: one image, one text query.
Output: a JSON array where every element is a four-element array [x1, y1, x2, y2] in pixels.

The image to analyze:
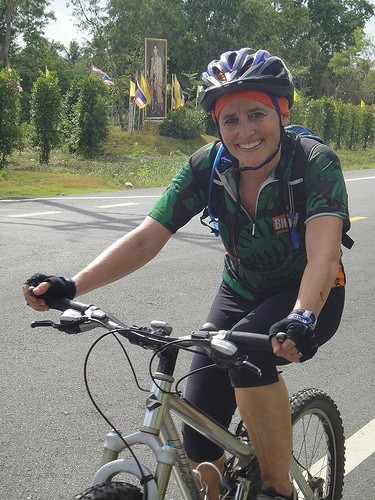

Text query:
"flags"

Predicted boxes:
[[294, 90, 300, 103], [171, 77, 185, 111], [360, 100, 367, 111], [130, 77, 151, 110], [91, 64, 115, 86], [8, 65, 23, 93], [45, 68, 55, 80], [197, 84, 204, 97]]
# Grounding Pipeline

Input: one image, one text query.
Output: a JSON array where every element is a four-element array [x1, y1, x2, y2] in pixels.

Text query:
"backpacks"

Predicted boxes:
[[207, 125, 354, 262]]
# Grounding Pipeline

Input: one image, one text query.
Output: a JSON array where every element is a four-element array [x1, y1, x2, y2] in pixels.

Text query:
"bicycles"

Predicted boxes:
[[26, 281, 346, 500]]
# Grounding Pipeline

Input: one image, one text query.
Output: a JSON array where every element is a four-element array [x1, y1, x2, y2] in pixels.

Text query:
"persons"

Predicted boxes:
[[23, 47, 357, 500]]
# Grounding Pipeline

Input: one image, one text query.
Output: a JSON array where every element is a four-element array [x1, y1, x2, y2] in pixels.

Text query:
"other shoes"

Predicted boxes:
[[257, 483, 298, 500]]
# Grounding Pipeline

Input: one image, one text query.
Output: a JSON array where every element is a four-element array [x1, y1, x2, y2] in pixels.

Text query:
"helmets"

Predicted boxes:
[[200, 47, 294, 113]]
